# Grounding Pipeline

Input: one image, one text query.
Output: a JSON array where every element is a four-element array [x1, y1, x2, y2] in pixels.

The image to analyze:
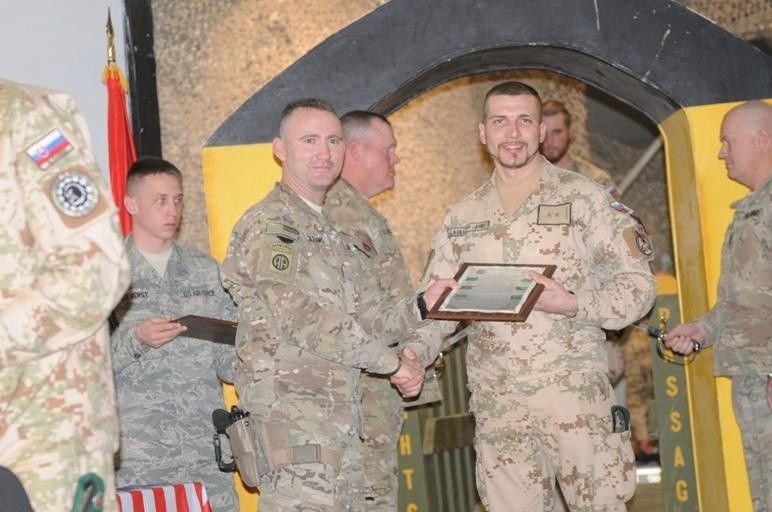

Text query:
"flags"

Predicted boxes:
[[99, 62, 142, 245]]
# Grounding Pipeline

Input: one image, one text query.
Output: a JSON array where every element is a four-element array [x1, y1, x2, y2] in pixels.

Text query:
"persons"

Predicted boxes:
[[105, 156, 242, 512], [660, 99, 770, 512], [320, 107, 454, 512], [537, 99, 629, 416], [218, 96, 462, 512], [388, 81, 660, 512], [0, 76, 134, 511]]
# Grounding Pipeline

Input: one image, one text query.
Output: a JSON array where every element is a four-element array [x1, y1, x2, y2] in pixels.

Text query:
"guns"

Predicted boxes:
[[213, 406, 246, 432]]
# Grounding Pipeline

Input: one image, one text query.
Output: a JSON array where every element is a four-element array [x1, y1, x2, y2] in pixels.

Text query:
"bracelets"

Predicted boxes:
[[416, 291, 430, 322], [390, 356, 404, 375]]
